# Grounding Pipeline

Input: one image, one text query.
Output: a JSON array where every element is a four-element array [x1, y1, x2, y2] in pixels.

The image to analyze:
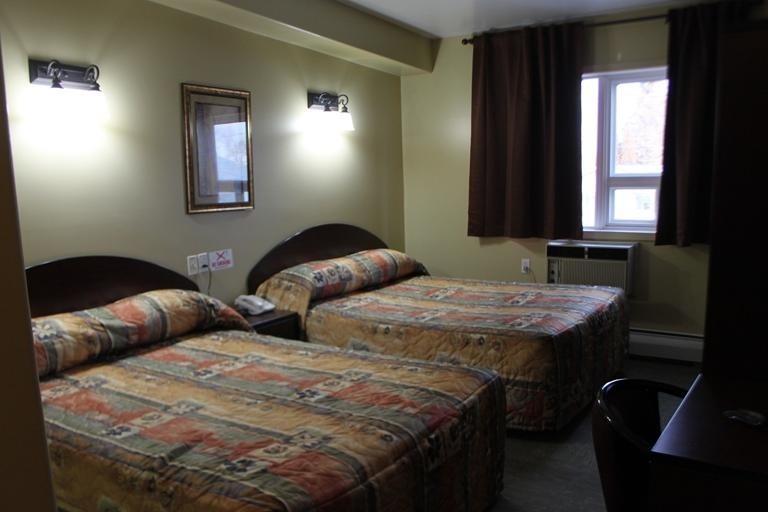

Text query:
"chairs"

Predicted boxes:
[[592, 378, 688, 512]]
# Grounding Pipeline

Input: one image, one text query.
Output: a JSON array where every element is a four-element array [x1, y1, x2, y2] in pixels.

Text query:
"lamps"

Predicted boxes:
[[29, 58, 102, 115], [307, 91, 354, 131]]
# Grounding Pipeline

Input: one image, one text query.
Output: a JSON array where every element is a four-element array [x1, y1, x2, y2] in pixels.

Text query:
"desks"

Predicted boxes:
[[649, 374, 767, 512]]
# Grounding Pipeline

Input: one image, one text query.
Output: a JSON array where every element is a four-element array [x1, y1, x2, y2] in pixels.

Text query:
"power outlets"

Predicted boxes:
[[197, 252, 208, 273], [521, 258, 529, 273], [187, 255, 198, 276]]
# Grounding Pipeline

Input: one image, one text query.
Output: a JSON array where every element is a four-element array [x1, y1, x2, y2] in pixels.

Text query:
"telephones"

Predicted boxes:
[[234, 294, 276, 316]]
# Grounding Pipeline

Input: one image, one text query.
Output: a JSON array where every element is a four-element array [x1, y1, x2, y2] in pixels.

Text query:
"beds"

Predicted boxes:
[[23, 255, 506, 512], [245, 222, 629, 432]]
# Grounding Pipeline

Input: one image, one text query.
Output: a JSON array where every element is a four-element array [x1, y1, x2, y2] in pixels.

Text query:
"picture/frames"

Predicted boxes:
[[180, 82, 254, 214]]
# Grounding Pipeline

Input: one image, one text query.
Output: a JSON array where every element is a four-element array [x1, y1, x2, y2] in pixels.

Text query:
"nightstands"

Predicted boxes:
[[226, 303, 298, 340]]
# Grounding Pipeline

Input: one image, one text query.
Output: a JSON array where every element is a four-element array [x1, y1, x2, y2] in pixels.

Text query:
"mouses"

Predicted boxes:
[[720, 406, 765, 431]]
